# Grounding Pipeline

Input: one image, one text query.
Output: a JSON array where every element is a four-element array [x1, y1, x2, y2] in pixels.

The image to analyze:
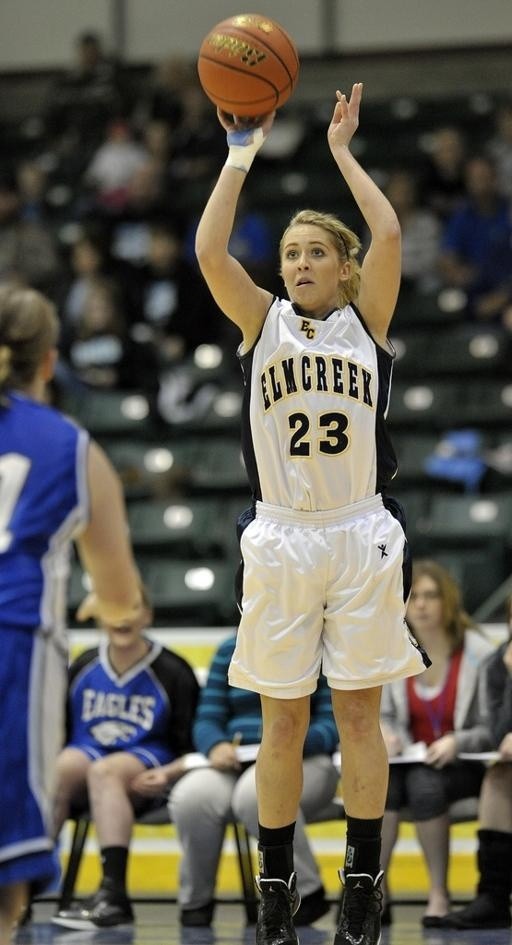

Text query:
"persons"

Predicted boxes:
[[441, 594, 512, 929], [18, 588, 200, 926], [194, 83, 432, 945], [166, 636, 341, 927], [0, 33, 511, 497], [0, 278, 145, 945], [379, 560, 496, 926]]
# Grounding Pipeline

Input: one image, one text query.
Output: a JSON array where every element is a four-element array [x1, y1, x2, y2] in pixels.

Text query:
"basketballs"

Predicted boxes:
[[198, 12, 300, 117]]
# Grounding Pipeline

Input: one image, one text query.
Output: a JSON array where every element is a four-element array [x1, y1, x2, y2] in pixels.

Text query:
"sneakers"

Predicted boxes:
[[254, 872, 329, 945], [51, 880, 133, 930], [334, 869, 384, 945], [181, 898, 214, 927], [422, 894, 512, 929]]
